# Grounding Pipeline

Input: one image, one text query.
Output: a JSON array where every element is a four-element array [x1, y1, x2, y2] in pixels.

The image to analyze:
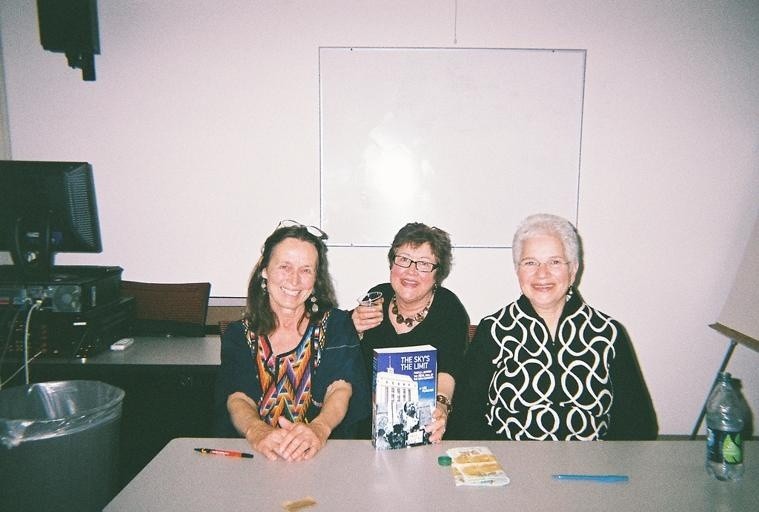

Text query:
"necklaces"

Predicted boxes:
[[390, 291, 432, 327]]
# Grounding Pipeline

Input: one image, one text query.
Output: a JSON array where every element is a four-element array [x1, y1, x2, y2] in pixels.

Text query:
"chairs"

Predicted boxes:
[[120, 279, 211, 338]]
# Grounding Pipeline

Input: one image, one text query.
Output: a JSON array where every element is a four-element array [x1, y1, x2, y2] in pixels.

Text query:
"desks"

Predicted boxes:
[[101, 434, 758, 512]]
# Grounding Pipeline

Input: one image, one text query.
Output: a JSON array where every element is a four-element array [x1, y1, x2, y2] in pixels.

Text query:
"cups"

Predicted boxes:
[[357, 291, 384, 320]]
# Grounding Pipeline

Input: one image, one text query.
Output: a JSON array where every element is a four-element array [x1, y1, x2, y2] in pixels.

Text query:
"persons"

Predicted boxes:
[[339, 222, 471, 445], [460, 215, 659, 441], [221, 225, 360, 460]]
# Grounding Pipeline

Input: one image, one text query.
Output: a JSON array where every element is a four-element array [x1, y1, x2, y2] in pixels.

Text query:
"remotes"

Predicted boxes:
[[111, 338, 135, 351]]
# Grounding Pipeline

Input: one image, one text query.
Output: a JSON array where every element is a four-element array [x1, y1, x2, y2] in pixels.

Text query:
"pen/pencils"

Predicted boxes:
[[553, 474, 629, 481], [194, 447, 255, 459]]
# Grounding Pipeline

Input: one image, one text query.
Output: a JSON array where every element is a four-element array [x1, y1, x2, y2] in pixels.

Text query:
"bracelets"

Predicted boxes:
[[437, 394, 455, 412]]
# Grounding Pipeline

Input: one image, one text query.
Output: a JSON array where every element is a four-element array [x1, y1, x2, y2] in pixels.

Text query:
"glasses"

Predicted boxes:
[[518, 255, 571, 270], [392, 253, 439, 274], [275, 218, 328, 247]]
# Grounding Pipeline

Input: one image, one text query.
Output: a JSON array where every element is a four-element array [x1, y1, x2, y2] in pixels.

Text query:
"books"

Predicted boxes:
[[371, 345, 441, 450]]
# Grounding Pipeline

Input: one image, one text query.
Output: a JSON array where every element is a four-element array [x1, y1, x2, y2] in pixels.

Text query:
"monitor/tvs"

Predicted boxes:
[[2, 156, 104, 284]]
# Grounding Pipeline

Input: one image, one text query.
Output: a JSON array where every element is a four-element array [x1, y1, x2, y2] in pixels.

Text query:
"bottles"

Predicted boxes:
[[705, 371, 747, 481]]
